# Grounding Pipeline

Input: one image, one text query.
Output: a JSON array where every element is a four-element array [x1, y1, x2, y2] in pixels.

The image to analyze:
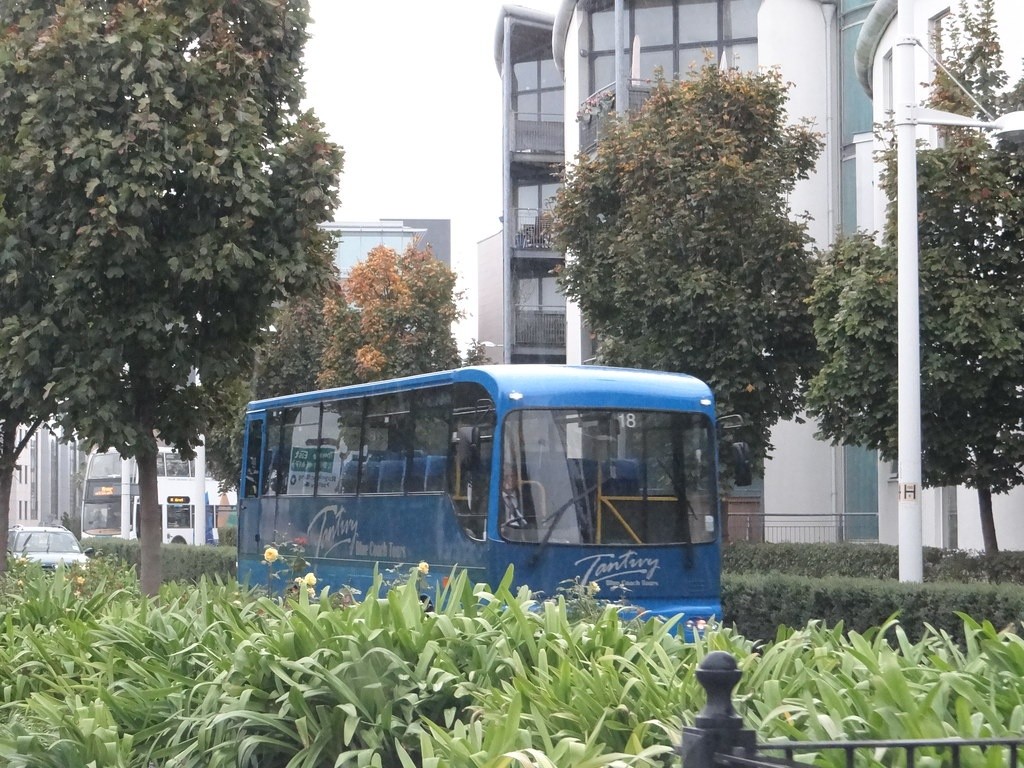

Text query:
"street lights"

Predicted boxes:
[[898, 1, 1024, 583]]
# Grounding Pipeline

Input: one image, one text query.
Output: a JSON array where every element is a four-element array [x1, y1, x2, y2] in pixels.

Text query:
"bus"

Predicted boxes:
[[80, 447, 237, 547], [237, 363, 725, 643]]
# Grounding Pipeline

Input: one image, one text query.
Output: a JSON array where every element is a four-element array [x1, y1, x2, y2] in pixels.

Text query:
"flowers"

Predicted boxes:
[[575, 88, 613, 122]]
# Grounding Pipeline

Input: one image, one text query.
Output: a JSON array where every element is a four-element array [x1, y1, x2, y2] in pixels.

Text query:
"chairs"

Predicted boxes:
[[340, 455, 490, 493], [517, 459, 639, 497]]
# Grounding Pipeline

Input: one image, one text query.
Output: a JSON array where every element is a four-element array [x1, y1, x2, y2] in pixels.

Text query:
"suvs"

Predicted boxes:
[[7, 524, 93, 572]]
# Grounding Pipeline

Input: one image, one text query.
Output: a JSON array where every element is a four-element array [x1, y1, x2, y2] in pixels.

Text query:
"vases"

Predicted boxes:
[[601, 100, 611, 110], [578, 114, 591, 121], [587, 108, 600, 116]]
